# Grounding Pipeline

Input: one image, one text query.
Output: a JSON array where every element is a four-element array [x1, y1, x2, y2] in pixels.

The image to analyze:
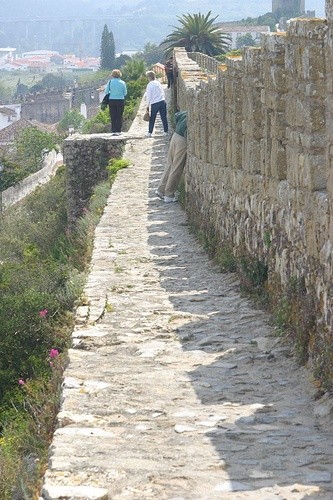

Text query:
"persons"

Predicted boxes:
[[145, 71, 168, 137], [165, 57, 173, 89], [155, 110, 188, 203], [104, 69, 127, 136]]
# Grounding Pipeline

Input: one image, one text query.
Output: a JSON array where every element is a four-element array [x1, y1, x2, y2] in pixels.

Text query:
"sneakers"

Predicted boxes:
[[155, 190, 163, 199], [163, 196, 178, 203]]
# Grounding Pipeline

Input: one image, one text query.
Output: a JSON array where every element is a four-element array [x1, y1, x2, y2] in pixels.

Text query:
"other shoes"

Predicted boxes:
[[113, 131, 122, 136], [145, 133, 151, 138], [163, 132, 168, 136]]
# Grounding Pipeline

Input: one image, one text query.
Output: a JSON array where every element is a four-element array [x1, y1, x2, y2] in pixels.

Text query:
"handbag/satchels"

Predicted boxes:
[[103, 94, 110, 104], [143, 110, 150, 121]]
